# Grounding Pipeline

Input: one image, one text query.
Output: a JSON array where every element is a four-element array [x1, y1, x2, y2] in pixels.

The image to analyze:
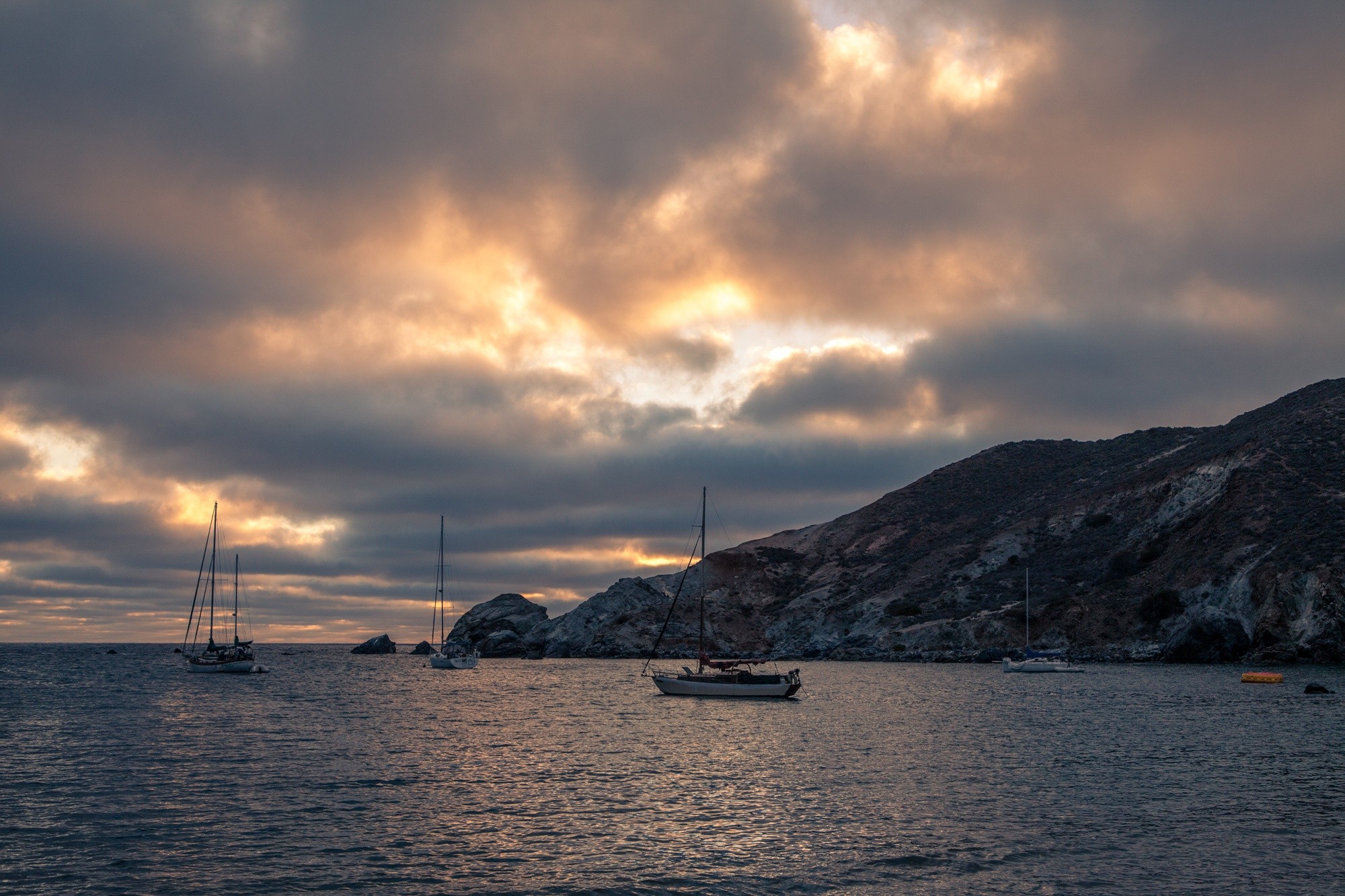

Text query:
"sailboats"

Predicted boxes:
[[429, 514, 481, 668], [184, 500, 256, 672], [642, 485, 803, 697], [1003, 567, 1085, 672]]
